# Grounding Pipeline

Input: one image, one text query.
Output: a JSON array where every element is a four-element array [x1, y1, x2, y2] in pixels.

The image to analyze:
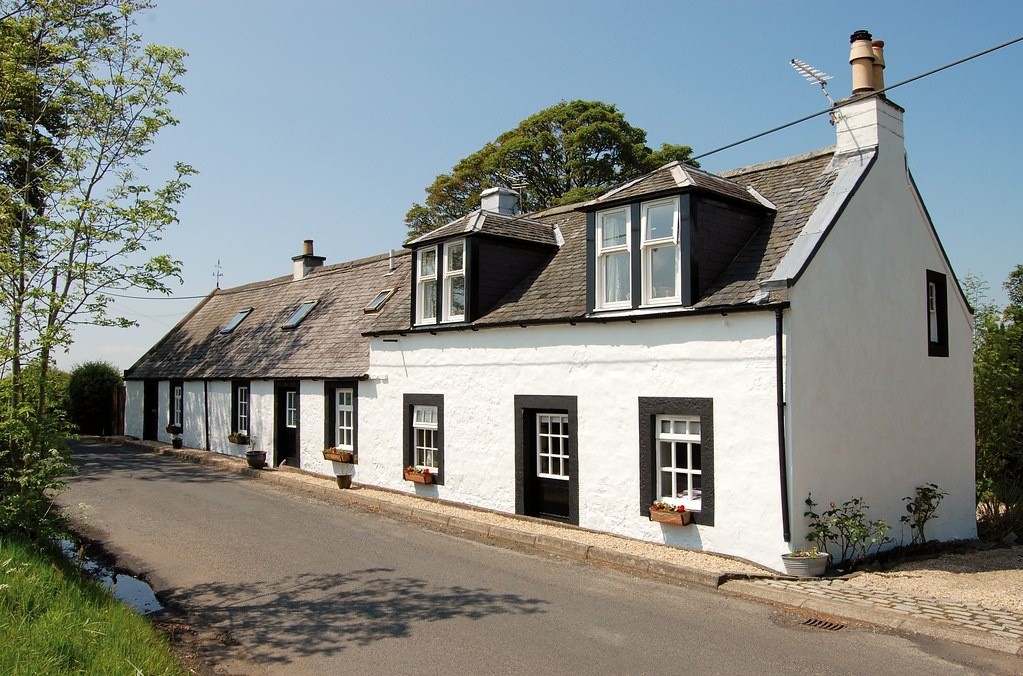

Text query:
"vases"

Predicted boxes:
[[649, 508, 691, 528], [404, 473, 433, 484], [322, 451, 350, 463], [781, 553, 829, 576], [336, 474, 353, 490]]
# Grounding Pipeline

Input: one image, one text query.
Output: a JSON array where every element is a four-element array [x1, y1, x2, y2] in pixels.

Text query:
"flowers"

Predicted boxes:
[[403, 465, 430, 475], [790, 547, 819, 557], [324, 447, 346, 454], [652, 501, 686, 513]]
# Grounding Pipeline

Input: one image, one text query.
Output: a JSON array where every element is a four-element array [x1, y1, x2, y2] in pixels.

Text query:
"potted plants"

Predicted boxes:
[[227, 432, 251, 445], [166, 424, 181, 435], [171, 437, 183, 449], [245, 435, 267, 468]]
